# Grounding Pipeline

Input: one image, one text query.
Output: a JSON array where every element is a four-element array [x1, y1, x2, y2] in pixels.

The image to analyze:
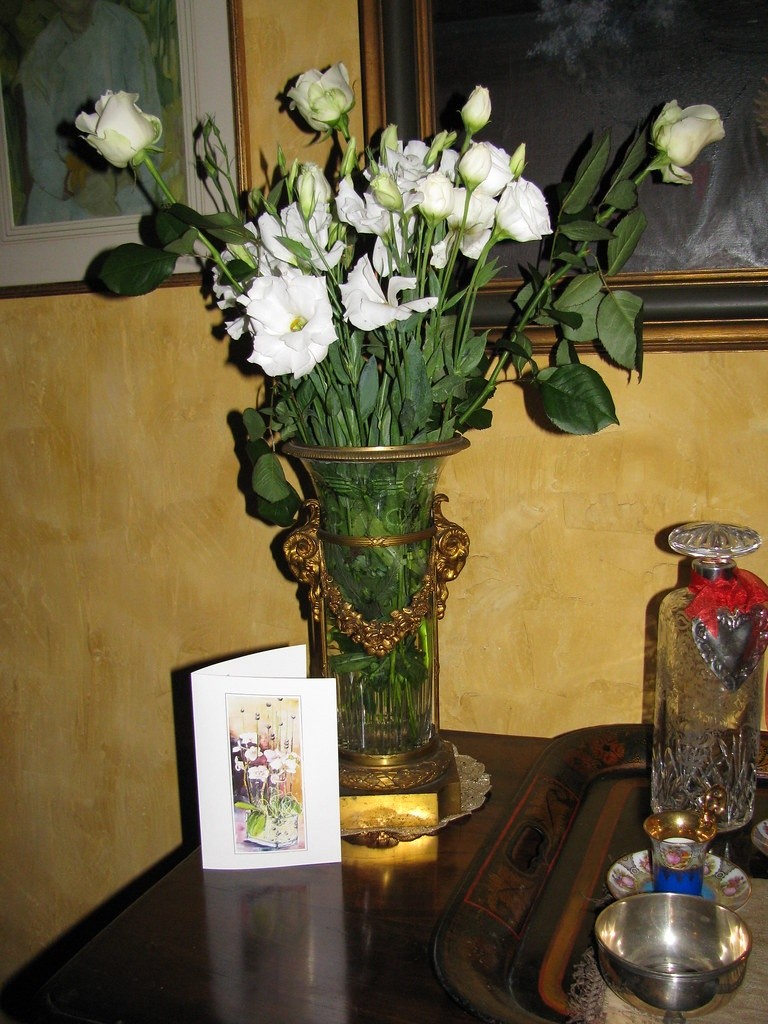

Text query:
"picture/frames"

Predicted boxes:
[[0, 0, 257, 301]]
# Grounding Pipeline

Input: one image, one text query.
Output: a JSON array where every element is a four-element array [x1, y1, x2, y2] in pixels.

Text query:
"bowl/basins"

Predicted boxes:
[[593, 894, 751, 1012]]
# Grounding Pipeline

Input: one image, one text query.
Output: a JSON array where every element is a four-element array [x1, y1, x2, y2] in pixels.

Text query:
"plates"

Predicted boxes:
[[607, 850, 751, 912], [751, 820, 768, 856]]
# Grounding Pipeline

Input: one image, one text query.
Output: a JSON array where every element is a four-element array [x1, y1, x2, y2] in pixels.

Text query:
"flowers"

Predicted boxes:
[[69, 58, 726, 751]]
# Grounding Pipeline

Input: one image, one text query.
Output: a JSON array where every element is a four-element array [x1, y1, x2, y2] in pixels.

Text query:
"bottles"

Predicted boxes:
[[652, 521, 768, 833]]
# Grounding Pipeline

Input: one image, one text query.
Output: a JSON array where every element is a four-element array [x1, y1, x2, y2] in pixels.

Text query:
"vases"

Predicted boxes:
[[355, 0, 768, 356], [277, 427, 471, 842]]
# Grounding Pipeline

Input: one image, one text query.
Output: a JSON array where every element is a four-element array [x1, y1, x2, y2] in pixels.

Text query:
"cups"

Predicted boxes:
[[643, 785, 727, 896]]
